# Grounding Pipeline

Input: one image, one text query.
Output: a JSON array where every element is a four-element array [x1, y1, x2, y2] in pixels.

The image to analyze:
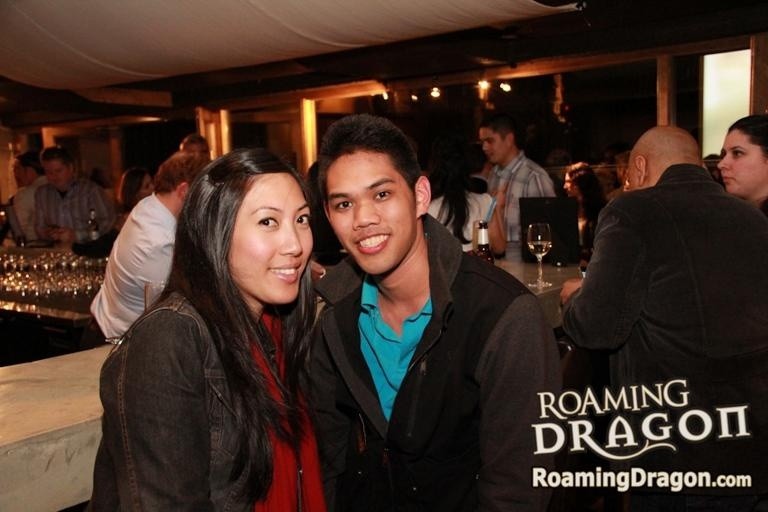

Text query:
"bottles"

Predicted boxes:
[[477, 221, 495, 264], [88, 209, 100, 240]]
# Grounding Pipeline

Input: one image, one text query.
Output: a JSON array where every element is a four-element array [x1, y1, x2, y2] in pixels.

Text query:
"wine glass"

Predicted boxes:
[[527, 223, 553, 288], [0, 250, 107, 305]]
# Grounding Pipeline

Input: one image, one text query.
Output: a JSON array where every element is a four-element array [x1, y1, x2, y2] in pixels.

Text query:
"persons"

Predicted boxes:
[[303, 159, 345, 268], [297, 110, 564, 511], [421, 112, 767, 262], [560, 125, 767, 509], [2, 130, 215, 349], [85, 142, 347, 512]]
